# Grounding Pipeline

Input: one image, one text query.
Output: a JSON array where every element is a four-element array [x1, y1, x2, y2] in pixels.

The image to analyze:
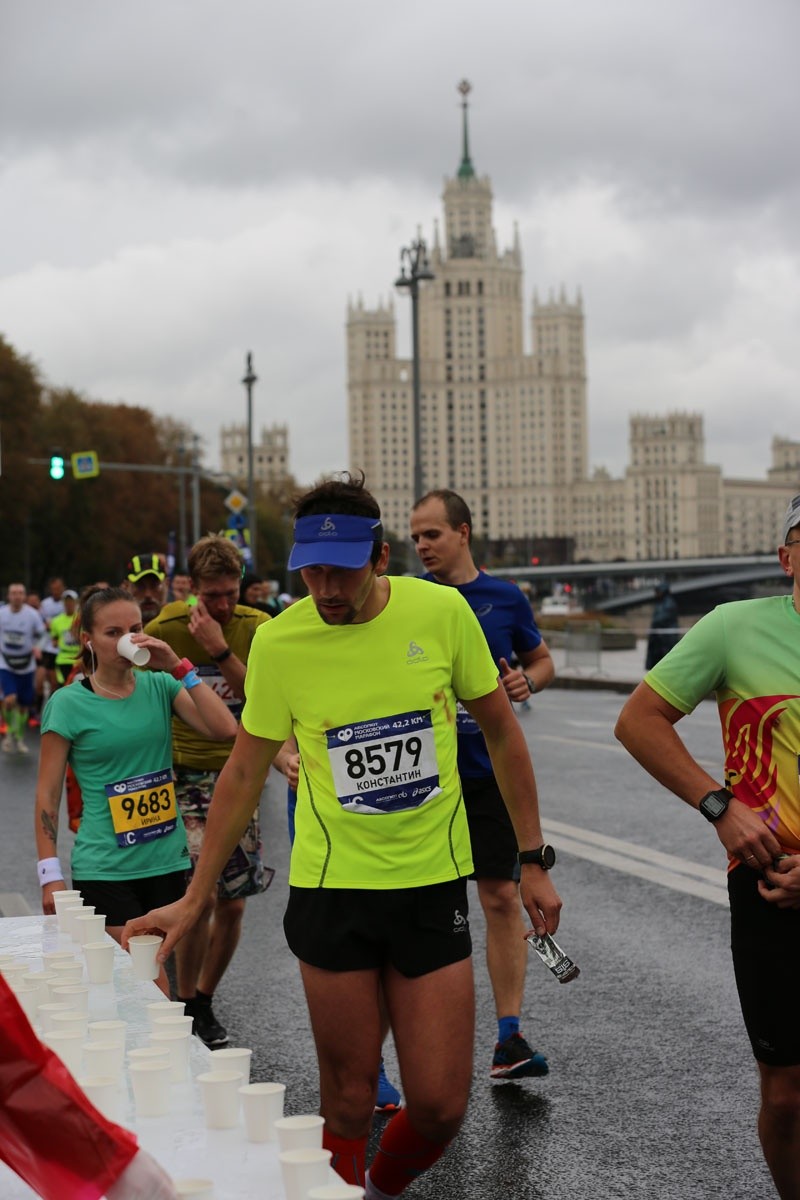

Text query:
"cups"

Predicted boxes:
[[237, 1082, 286, 1144], [278, 1148, 333, 1200], [116, 632, 151, 667], [274, 1113, 325, 1149], [306, 1184, 366, 1200], [0, 889, 252, 1136], [172, 1178, 215, 1200]]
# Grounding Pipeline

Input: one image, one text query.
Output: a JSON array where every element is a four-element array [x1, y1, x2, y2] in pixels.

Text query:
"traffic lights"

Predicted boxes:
[[50, 454, 66, 480]]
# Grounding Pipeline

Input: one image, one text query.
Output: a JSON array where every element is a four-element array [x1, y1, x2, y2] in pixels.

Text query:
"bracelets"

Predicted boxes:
[[210, 648, 230, 663], [171, 657, 195, 680], [181, 671, 202, 689], [523, 672, 537, 694], [37, 857, 65, 888]]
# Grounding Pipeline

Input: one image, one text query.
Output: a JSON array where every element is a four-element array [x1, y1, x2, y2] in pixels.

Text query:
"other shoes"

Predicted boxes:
[[16, 737, 30, 754], [193, 1006, 230, 1045]]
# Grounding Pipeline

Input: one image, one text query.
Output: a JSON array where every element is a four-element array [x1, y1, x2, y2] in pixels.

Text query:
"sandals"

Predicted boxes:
[[2, 735, 14, 752]]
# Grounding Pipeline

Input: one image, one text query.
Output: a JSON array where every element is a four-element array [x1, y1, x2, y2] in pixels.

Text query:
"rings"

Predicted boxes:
[[746, 854, 755, 860]]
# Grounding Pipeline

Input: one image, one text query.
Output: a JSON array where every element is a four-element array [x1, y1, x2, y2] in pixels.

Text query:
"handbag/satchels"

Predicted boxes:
[[2, 651, 33, 669]]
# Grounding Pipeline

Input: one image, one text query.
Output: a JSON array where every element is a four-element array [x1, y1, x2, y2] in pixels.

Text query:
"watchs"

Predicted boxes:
[[698, 786, 736, 825], [516, 844, 556, 871]]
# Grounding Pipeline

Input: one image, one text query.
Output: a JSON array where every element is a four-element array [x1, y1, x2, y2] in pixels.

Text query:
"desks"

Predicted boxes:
[[0, 914, 345, 1200]]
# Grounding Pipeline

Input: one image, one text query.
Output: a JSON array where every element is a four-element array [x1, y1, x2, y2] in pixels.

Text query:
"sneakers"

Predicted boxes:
[[488, 1031, 549, 1084], [374, 1056, 402, 1114]]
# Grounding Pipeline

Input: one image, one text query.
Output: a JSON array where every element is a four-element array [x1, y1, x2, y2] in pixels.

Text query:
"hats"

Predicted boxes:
[[62, 589, 79, 600], [127, 554, 165, 584], [783, 496, 800, 546], [286, 513, 382, 572]]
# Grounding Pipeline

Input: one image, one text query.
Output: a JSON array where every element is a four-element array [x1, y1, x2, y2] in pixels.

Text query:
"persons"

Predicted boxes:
[[121, 469, 562, 1200], [645, 581, 680, 671], [0, 468, 553, 1117], [614, 493, 800, 1200]]
